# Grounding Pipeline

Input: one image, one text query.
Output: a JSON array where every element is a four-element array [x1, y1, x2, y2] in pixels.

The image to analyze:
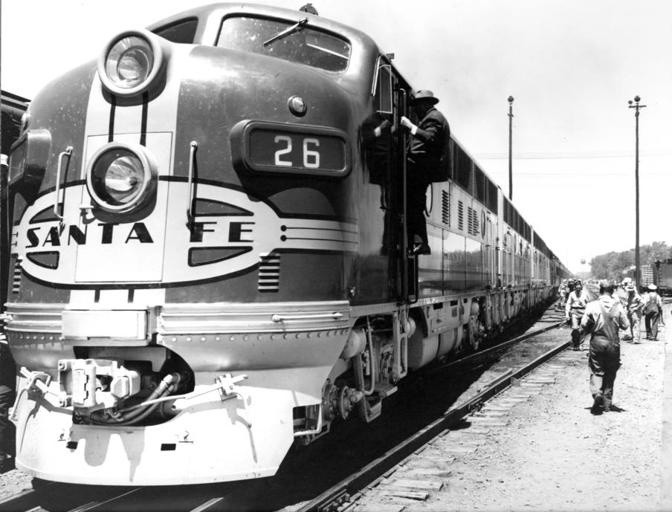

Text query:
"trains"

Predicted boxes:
[[0, 88, 34, 476], [1, 3, 581, 497]]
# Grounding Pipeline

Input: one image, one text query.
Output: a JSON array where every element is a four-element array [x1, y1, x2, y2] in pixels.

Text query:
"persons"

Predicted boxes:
[[557, 275, 666, 352], [580, 281, 631, 415]]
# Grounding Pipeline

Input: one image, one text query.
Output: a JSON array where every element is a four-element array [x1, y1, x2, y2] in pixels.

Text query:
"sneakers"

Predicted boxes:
[[592, 393, 604, 413]]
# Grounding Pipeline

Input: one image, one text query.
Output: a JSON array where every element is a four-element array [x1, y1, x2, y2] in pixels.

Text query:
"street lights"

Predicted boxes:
[[504, 95, 515, 203], [627, 95, 646, 289]]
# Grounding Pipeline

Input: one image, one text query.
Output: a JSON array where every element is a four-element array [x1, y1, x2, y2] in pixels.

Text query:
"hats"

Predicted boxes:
[[599, 279, 613, 288], [408, 90, 440, 105], [648, 283, 658, 291]]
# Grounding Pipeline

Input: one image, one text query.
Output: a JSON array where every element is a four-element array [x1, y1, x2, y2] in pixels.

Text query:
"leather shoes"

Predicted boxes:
[[407, 242, 431, 254]]
